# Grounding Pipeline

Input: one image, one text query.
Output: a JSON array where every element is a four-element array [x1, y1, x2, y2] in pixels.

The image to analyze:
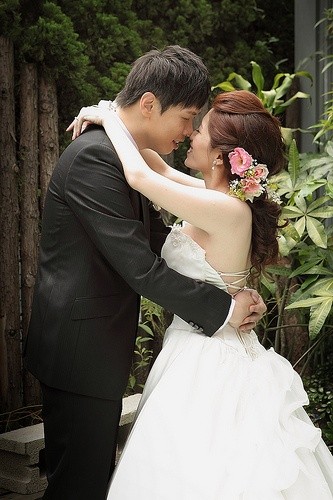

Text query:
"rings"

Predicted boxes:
[[75, 117, 79, 122]]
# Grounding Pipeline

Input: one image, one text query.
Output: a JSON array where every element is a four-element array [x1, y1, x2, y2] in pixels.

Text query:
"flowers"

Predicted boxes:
[[226, 146, 270, 203]]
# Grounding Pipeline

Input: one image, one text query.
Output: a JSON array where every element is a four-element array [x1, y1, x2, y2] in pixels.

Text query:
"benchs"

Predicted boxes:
[[0, 393, 143, 500]]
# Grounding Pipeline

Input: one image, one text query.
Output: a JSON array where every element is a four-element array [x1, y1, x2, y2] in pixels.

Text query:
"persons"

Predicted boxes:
[[22, 45, 266, 500], [65, 90, 333, 500]]
